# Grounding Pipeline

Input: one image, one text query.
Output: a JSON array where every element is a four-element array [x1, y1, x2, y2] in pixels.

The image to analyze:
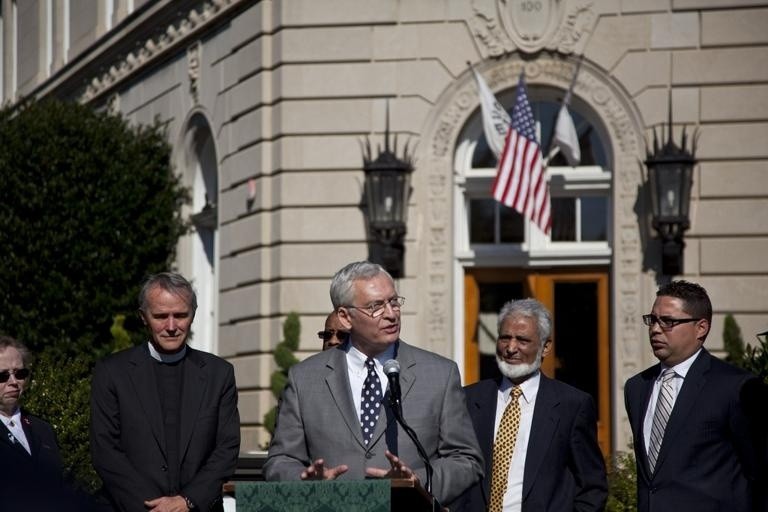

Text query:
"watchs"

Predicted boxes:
[[179, 492, 197, 509]]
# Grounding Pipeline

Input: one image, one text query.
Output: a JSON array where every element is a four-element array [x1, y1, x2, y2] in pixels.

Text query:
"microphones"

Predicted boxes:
[[383, 359, 402, 419]]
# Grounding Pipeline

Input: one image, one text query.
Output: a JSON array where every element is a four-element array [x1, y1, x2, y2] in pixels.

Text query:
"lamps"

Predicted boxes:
[[640, 84, 701, 275], [356, 92, 417, 277]]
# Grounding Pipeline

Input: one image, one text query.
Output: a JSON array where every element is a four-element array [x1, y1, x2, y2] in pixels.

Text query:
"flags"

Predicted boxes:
[[548, 105, 582, 168], [492, 70, 554, 236], [467, 61, 514, 164]]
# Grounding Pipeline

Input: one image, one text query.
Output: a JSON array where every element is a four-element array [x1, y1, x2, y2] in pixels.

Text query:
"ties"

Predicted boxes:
[[647, 369, 677, 475], [488, 384, 522, 511], [360, 356, 382, 446]]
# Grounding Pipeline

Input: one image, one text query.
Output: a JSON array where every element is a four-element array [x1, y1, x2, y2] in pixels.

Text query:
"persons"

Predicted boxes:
[[454, 293, 607, 511], [624, 280, 768, 511], [263, 260, 488, 507], [88, 271, 241, 511], [1, 335, 68, 511], [314, 306, 351, 352]]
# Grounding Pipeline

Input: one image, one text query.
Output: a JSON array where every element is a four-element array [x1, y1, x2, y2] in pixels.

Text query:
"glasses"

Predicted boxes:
[[0, 368, 30, 383], [642, 314, 701, 330], [318, 330, 350, 340], [335, 296, 406, 319]]
